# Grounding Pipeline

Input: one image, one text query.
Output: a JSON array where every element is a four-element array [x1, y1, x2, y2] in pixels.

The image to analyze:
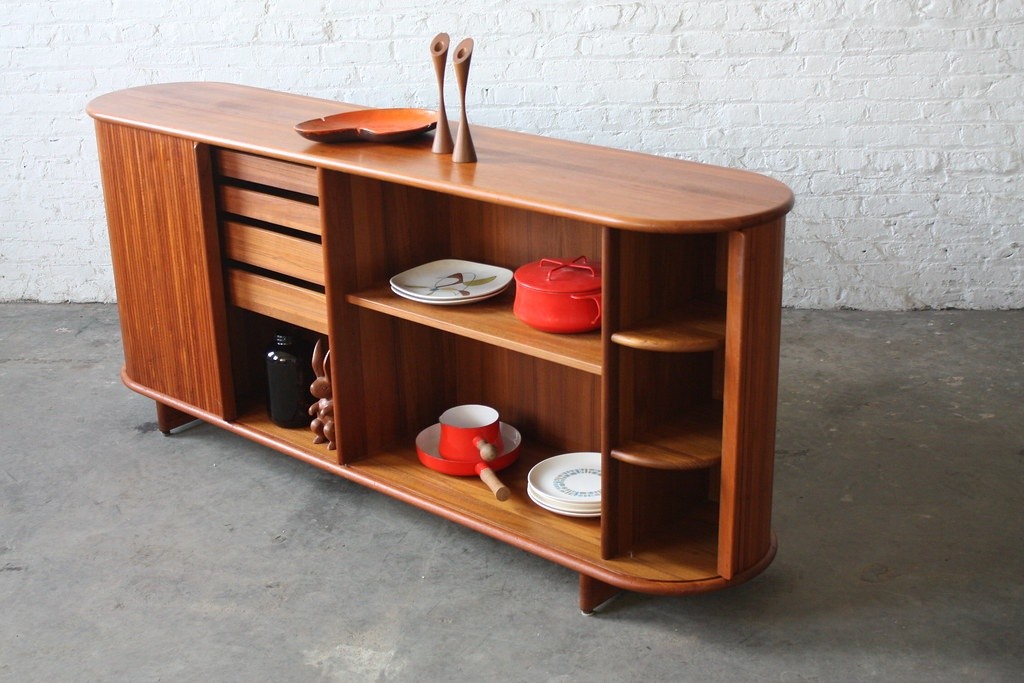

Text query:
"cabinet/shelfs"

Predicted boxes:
[[84, 82, 795, 617]]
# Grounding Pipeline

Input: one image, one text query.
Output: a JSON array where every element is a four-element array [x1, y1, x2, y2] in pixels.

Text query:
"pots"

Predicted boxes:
[[513, 256, 603, 336], [417, 404, 522, 501]]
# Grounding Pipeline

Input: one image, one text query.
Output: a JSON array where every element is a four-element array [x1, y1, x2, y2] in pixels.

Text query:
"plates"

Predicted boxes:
[[295, 108, 441, 143], [526, 452, 601, 517], [389, 258, 513, 305]]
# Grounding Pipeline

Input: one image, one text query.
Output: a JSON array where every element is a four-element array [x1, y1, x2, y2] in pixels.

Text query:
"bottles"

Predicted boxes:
[[265, 331, 311, 430]]
